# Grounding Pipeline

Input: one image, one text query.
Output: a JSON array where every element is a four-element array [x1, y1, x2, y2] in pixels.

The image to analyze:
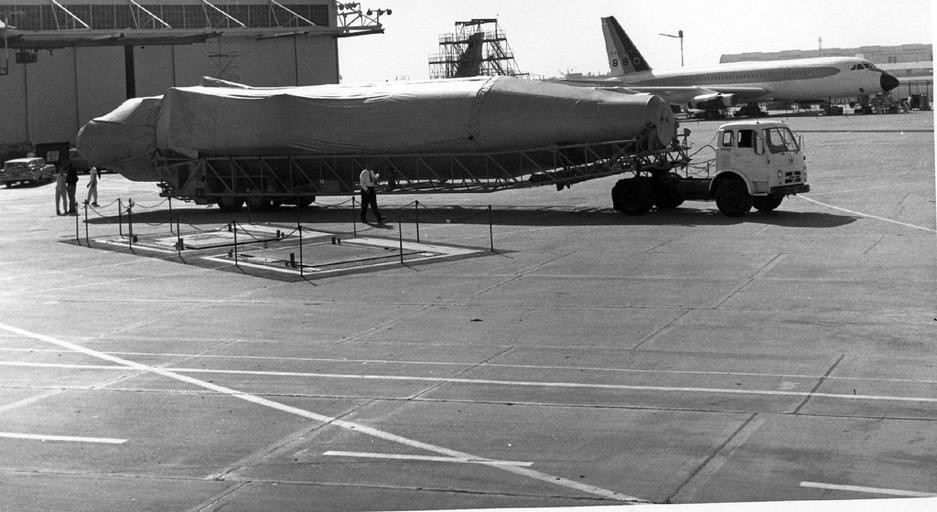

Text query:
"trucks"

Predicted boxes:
[[609, 118, 810, 217]]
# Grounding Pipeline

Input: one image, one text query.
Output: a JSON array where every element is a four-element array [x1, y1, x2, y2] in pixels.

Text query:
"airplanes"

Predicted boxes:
[[563, 16, 900, 117]]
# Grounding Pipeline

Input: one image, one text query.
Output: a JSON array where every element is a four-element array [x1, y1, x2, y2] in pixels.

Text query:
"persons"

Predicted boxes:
[[81, 165, 98, 206], [52, 168, 67, 216], [358, 164, 382, 225], [64, 167, 79, 213]]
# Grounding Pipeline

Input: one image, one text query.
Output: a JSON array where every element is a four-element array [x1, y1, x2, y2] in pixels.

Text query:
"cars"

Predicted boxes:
[[0, 156, 57, 187]]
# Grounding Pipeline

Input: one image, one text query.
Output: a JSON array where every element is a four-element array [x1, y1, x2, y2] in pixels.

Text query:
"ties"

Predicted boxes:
[[369, 170, 373, 182]]
